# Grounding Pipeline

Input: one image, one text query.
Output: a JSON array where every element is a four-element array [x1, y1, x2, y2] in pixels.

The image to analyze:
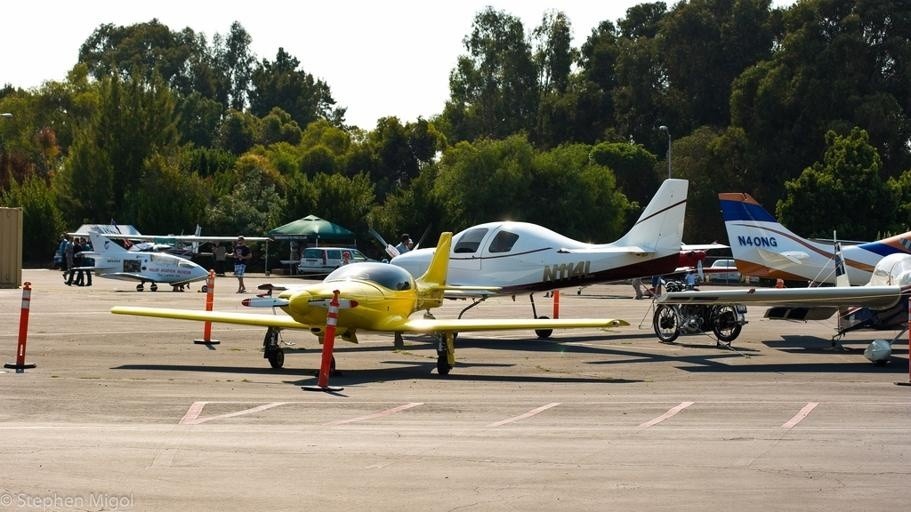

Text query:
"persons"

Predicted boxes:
[[631, 277, 643, 299], [684, 267, 697, 286], [407, 238, 414, 249], [341, 251, 352, 266], [58, 232, 92, 287], [395, 233, 410, 255], [651, 274, 662, 297], [224, 236, 252, 293]]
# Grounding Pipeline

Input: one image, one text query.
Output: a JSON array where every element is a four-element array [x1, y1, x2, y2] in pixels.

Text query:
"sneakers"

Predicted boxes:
[[63, 274, 91, 286]]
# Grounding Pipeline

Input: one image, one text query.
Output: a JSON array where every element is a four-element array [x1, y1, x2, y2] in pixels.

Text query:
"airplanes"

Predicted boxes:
[[58, 176, 911, 379]]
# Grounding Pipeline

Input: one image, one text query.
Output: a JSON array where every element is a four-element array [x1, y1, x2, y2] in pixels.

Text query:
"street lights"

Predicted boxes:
[[1, 112, 14, 120], [660, 123, 673, 178]]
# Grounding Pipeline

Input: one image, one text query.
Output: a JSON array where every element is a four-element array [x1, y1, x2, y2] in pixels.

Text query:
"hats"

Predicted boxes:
[[409, 239, 414, 244]]
